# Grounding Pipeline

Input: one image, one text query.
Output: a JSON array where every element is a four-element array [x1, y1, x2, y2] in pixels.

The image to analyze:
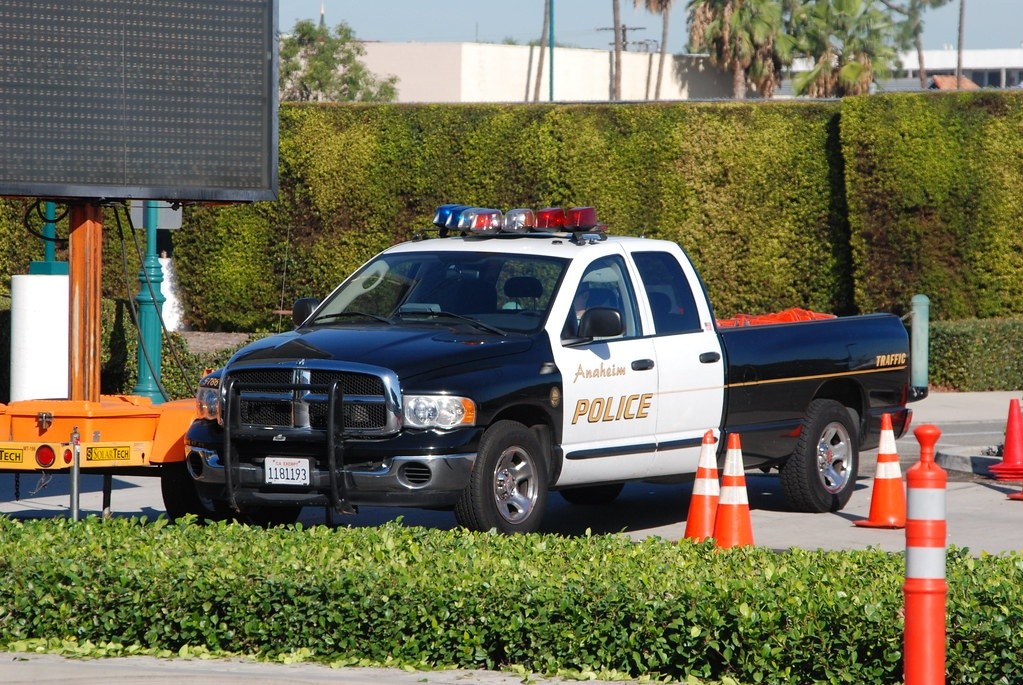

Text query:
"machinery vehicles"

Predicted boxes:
[[0, 200, 222, 519]]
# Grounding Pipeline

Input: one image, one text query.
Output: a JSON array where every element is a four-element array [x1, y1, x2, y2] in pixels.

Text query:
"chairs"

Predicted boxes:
[[503, 277, 542, 311], [572, 288, 620, 327]]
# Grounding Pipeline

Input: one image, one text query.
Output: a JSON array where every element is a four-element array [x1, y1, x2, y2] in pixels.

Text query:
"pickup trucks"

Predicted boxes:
[[184, 203, 914, 533]]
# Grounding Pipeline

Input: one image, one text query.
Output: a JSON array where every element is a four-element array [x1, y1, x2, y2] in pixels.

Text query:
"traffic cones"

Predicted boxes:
[[985, 399, 1023, 481], [712, 432, 755, 549], [684, 428, 720, 544], [853, 413, 907, 528]]
